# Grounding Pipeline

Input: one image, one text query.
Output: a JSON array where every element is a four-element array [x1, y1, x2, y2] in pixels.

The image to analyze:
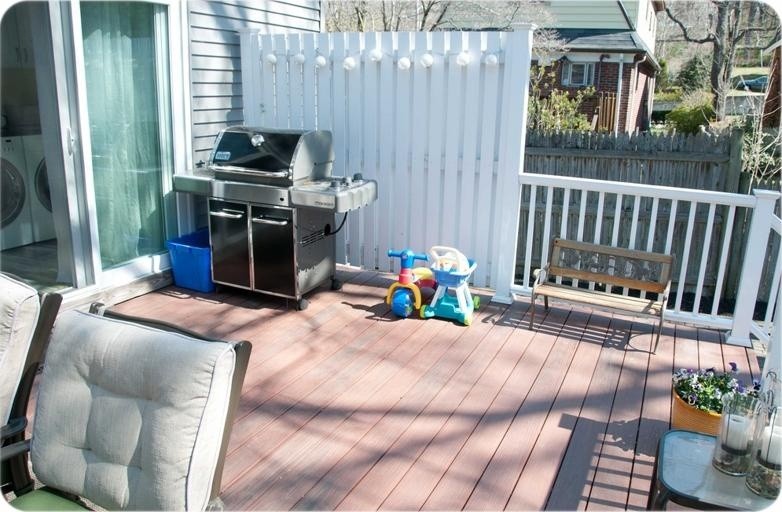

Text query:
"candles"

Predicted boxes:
[[722, 413, 752, 452], [760, 425, 781, 468]]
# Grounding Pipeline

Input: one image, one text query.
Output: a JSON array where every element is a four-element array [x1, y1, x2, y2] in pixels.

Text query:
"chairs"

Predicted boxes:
[[0, 270, 63, 498], [0, 300, 253, 511]]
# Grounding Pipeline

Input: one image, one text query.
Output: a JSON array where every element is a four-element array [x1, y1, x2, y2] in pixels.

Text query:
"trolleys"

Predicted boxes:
[[166, 121, 382, 315]]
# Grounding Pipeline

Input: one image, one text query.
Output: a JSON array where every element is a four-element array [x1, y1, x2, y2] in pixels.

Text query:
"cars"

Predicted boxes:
[[736, 72, 769, 93]]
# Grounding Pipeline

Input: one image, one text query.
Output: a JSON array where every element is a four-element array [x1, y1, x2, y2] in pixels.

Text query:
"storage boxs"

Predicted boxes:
[[162, 226, 215, 292]]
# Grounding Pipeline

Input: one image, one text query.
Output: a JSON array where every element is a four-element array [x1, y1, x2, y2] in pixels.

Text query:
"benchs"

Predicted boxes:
[[528, 237, 676, 356]]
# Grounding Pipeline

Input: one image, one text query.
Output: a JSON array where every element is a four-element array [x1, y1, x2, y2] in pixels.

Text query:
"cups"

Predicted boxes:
[[712, 389, 782, 500]]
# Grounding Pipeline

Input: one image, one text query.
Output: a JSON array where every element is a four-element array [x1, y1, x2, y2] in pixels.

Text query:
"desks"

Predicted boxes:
[[645, 427, 782, 511]]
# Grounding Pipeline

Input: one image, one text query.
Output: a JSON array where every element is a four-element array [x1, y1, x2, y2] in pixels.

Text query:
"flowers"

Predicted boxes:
[[671, 360, 768, 417]]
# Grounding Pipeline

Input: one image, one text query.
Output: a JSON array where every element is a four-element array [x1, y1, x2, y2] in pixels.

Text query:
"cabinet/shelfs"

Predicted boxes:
[[3, 5, 36, 69], [206, 196, 337, 301]]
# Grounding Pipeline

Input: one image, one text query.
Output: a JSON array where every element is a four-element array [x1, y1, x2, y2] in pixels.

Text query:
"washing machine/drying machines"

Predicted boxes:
[[0, 126, 57, 251]]
